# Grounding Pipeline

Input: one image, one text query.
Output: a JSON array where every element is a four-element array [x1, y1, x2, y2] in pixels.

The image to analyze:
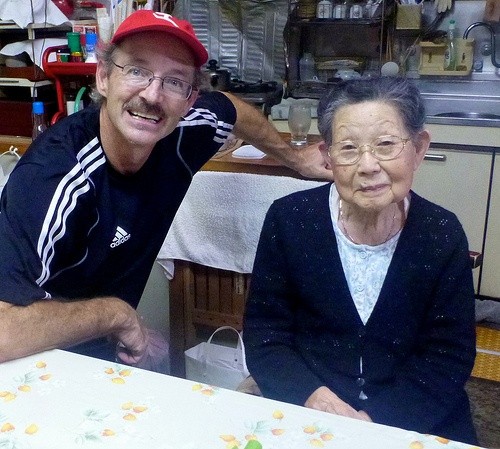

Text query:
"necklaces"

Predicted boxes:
[[339, 197, 403, 247]]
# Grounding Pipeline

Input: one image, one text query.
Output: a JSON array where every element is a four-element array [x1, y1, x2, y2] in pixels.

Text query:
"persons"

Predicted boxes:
[[242, 78, 477, 446], [0, 10, 336, 364]]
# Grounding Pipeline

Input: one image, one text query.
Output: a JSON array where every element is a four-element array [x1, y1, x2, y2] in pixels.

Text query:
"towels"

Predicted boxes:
[[155, 169, 330, 281]]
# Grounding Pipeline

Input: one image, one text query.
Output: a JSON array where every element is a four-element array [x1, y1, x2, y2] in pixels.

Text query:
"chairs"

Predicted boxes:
[[235, 249, 484, 400]]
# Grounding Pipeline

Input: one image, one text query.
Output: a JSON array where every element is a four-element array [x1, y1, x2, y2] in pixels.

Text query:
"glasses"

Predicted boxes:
[[110, 56, 198, 100], [327, 135, 413, 165]]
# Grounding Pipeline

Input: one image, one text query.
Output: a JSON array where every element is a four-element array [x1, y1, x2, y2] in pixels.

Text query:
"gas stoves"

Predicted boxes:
[[225, 81, 283, 111]]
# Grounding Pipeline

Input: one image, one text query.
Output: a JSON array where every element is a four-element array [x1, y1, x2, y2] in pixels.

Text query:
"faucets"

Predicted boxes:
[[463, 21, 500, 68]]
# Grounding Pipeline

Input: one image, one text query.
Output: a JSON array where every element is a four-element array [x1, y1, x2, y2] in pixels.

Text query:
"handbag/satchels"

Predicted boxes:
[[184, 326, 251, 392]]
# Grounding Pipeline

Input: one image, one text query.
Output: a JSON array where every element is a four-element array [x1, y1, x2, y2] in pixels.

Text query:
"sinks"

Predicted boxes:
[[420, 92, 500, 122]]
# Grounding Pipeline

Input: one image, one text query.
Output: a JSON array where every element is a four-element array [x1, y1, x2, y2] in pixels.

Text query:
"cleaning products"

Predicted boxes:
[[443, 19, 456, 71]]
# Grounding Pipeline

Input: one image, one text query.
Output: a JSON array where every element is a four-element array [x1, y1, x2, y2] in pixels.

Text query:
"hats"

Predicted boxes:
[[111, 9, 208, 71]]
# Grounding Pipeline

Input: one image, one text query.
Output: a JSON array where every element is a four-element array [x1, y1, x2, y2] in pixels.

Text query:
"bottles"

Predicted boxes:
[[315, 0, 333, 18], [299, 52, 315, 80], [56, 31, 97, 64], [32, 101, 47, 142], [443, 19, 457, 71]]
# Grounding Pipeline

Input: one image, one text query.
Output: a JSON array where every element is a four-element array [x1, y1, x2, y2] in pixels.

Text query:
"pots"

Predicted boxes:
[[206, 59, 230, 91]]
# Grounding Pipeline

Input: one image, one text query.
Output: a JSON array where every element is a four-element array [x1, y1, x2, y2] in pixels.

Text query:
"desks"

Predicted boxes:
[[0, 347, 489, 449], [153, 132, 330, 380], [41, 44, 97, 126]]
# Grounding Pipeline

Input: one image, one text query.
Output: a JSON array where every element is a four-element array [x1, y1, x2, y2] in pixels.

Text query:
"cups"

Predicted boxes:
[[333, 4, 350, 18], [287, 102, 312, 149], [350, 4, 363, 18]]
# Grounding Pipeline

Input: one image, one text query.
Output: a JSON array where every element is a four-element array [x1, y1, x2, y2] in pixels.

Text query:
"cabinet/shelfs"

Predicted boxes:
[[0, 17, 96, 139], [406, 146, 494, 296], [477, 151, 500, 301], [282, 0, 399, 99]]
